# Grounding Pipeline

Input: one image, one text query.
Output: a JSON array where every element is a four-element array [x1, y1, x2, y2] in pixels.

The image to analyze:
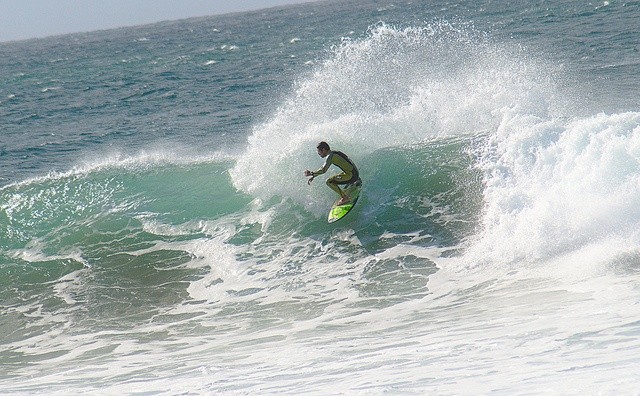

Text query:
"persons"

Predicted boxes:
[[304, 141, 360, 206]]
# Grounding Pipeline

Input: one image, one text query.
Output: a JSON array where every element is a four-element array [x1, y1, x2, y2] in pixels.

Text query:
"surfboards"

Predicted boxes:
[[328, 176, 362, 223]]
